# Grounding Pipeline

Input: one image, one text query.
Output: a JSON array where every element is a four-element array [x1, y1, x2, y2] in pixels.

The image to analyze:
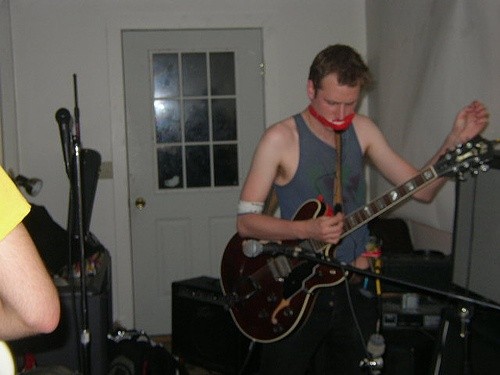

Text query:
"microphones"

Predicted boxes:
[[54, 107, 73, 176], [242, 239, 311, 262]]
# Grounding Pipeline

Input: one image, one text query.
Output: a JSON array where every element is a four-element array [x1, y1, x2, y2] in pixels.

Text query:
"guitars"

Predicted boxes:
[[219, 136, 495, 345]]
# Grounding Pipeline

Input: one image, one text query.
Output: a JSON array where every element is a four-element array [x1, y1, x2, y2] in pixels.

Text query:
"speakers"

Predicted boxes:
[[171, 274, 263, 375]]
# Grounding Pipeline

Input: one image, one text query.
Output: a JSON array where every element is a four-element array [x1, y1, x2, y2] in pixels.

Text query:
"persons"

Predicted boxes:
[[0, 165, 61, 342], [236, 43, 490, 375]]
